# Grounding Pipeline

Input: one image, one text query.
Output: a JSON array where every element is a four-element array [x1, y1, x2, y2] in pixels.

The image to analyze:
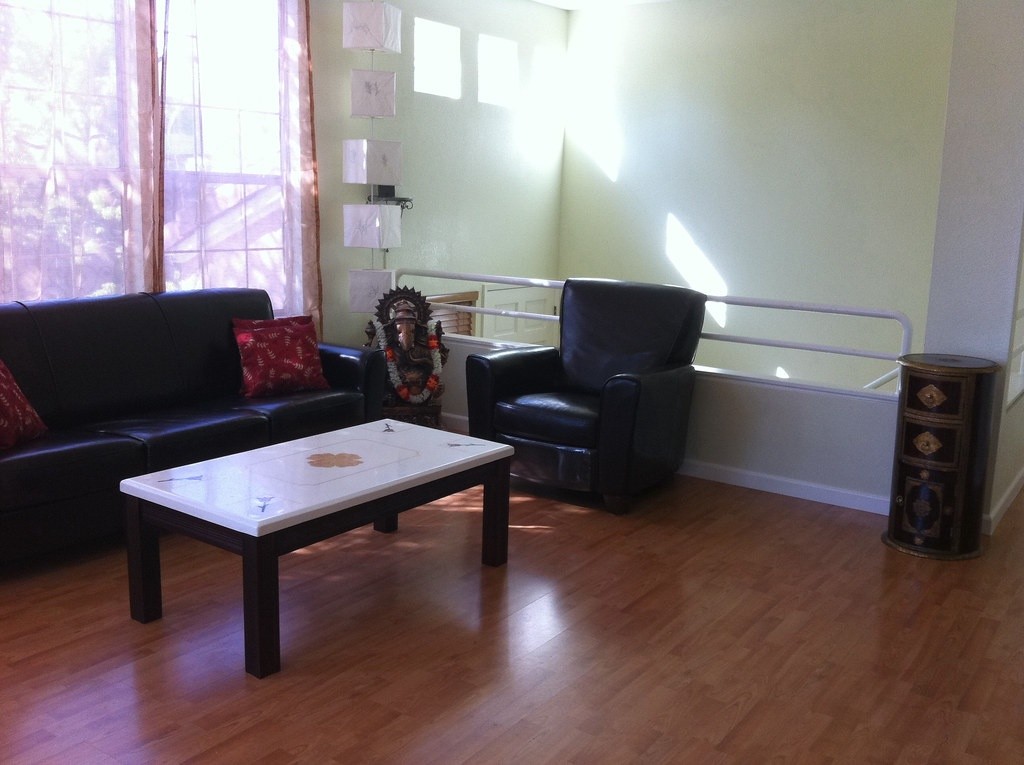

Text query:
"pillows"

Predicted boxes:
[[233, 321, 333, 398], [0, 359, 49, 452], [233, 314, 314, 329]]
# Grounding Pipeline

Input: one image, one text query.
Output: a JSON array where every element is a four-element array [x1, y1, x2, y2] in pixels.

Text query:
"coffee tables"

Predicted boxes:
[[119, 418, 515, 678]]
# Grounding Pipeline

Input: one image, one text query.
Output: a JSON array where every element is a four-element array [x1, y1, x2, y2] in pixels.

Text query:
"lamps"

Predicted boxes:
[[341, 1, 414, 315]]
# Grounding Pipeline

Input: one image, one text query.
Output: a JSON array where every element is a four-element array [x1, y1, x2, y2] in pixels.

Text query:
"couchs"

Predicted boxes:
[[466, 277, 708, 517], [1, 287, 387, 568]]
